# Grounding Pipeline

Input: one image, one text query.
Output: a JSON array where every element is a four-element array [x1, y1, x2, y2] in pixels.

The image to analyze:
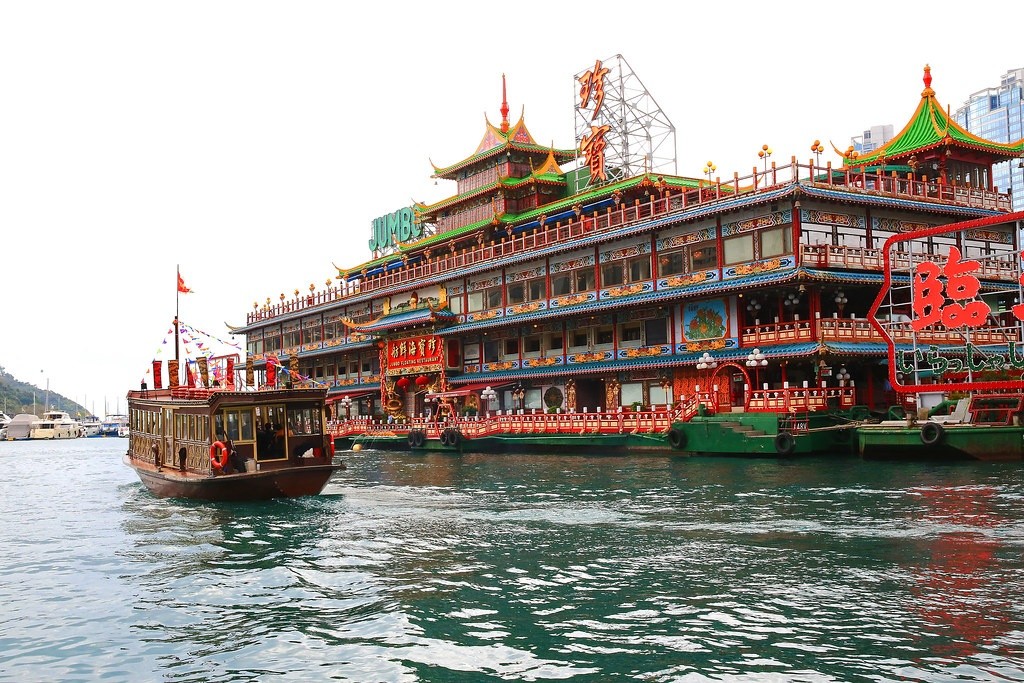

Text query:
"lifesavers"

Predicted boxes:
[[669, 429, 685, 449], [414, 431, 423, 447], [440, 432, 448, 445], [447, 430, 458, 446], [775, 432, 796, 456], [920, 422, 943, 446], [330, 432, 334, 456], [210, 441, 227, 468], [408, 431, 415, 446]]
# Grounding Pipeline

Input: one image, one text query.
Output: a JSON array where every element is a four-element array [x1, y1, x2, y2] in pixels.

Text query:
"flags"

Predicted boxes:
[[178, 272, 190, 292]]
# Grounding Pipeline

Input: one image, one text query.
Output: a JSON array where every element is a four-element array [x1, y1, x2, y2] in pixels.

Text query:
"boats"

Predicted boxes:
[[225, 53, 1024, 459], [29, 411, 80, 439], [70, 416, 101, 438], [101, 414, 129, 436], [0, 411, 11, 440], [123, 316, 347, 500]]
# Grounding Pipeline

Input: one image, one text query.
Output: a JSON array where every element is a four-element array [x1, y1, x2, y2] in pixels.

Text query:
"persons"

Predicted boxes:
[[388, 413, 392, 423], [215, 415, 308, 442], [883, 378, 892, 391]]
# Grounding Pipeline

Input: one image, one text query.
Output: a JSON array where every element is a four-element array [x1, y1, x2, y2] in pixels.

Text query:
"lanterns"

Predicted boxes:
[[416, 375, 429, 389], [397, 377, 410, 391]]
